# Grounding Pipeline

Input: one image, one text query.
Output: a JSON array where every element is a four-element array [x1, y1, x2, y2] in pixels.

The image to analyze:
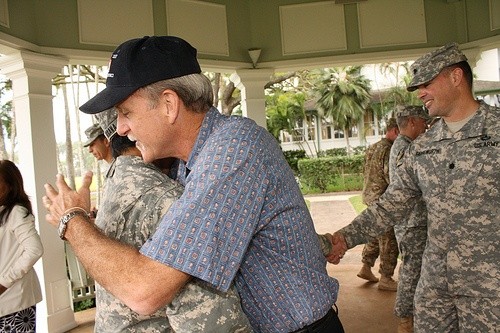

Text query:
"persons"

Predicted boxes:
[[357, 118, 400, 290], [0, 159, 44, 333], [326, 42, 500, 333], [83, 123, 115, 223], [389, 106, 431, 333], [42, 36, 343, 333], [317, 233, 347, 259], [93, 108, 255, 333]]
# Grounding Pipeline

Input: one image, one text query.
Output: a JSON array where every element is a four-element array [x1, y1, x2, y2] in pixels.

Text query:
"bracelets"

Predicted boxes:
[[61, 206, 91, 216]]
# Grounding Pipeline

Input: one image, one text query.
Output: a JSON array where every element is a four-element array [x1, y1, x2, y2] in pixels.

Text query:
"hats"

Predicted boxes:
[[407, 43, 467, 92], [95, 107, 118, 142], [387, 119, 398, 127], [395, 105, 430, 120], [79, 35, 201, 114], [82, 123, 104, 147]]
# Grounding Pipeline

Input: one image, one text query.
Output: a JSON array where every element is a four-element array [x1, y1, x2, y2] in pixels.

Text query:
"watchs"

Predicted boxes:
[[56, 210, 92, 242]]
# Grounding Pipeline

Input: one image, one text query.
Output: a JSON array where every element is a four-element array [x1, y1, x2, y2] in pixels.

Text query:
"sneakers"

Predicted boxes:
[[399, 316, 414, 333], [378, 275, 397, 291], [357, 263, 379, 282]]
